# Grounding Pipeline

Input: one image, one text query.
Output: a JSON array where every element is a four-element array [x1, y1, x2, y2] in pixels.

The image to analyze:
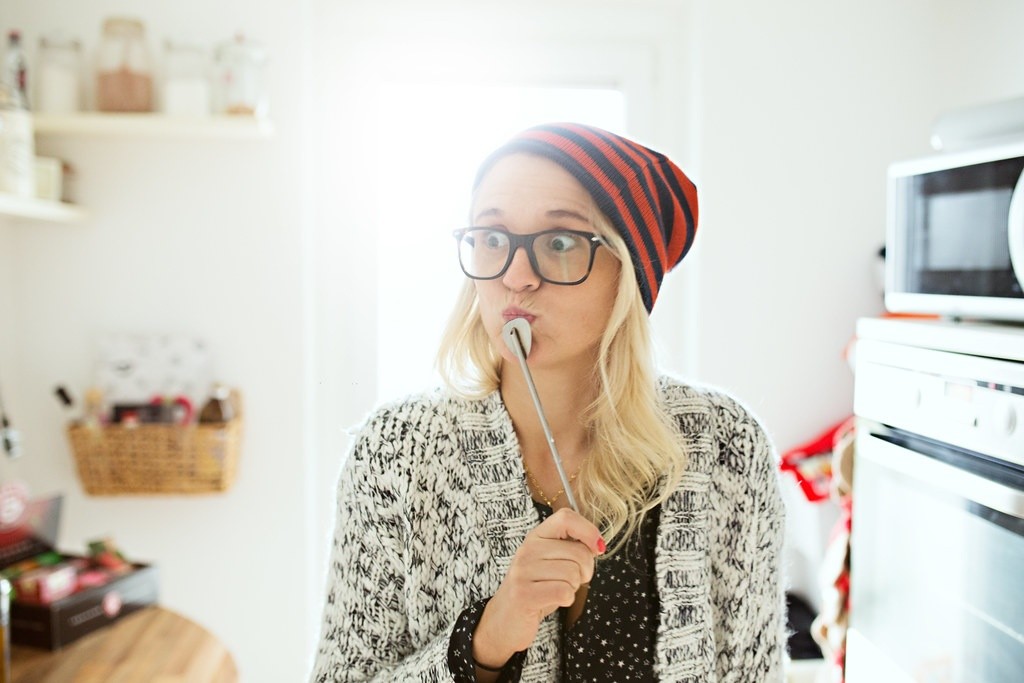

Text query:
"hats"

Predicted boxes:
[[469, 123, 700, 314]]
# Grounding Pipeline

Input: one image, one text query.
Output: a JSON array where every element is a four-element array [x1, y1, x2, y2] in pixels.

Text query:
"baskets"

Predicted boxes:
[[67, 415, 241, 497]]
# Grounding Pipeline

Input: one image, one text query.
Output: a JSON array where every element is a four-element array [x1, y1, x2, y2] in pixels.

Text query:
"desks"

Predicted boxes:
[[11, 607, 240, 683]]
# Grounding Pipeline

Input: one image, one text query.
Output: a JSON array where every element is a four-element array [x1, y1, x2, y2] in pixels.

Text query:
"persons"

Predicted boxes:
[[315, 122, 791, 682]]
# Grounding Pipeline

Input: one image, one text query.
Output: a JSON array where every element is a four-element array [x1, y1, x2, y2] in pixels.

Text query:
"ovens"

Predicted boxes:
[[845, 342, 1024, 683]]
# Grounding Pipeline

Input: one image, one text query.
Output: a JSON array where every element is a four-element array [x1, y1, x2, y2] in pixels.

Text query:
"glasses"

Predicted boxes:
[[452, 221, 610, 285]]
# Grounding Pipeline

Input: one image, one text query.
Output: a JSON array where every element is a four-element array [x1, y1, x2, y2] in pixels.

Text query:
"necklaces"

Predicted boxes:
[[525, 464, 582, 506]]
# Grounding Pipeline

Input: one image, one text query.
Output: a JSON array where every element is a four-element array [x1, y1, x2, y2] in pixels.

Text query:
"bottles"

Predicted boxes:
[[149, 396, 192, 423], [214, 34, 257, 116], [99, 16, 152, 111], [197, 384, 228, 483], [112, 362, 145, 427], [3, 32, 29, 109], [56, 386, 108, 431]]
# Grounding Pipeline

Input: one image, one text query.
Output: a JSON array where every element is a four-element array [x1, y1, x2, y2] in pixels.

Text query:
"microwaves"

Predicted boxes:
[[883, 141, 1024, 324]]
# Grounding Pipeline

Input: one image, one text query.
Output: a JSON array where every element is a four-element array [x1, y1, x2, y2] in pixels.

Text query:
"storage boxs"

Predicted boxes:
[[0, 479, 156, 650]]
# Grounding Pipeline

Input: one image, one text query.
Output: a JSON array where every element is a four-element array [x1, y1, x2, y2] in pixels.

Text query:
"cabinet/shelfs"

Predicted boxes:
[[0, 107, 278, 220]]
